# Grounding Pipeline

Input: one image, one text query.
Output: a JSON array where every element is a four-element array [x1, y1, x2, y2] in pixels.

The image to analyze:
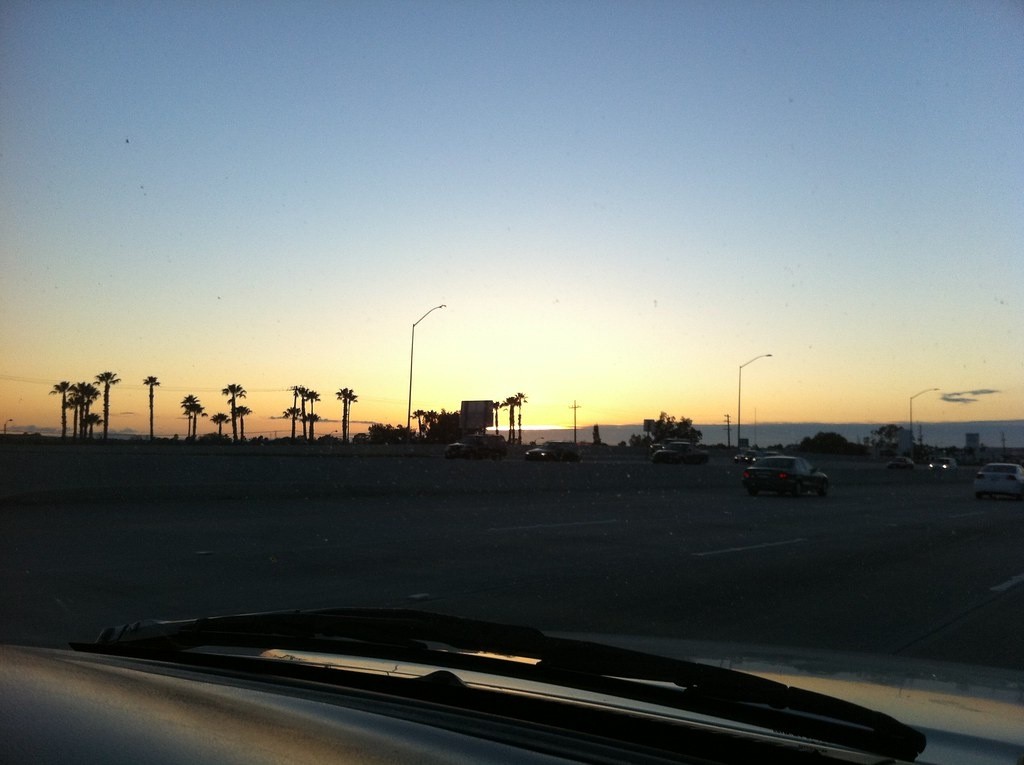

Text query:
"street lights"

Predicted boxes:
[[909, 387, 940, 458], [736, 352, 771, 453], [405, 304, 447, 445]]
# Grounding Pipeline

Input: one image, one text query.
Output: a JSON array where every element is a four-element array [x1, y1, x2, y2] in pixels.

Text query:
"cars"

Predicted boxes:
[[648, 437, 710, 466], [523, 439, 582, 464], [444, 432, 508, 461], [926, 456, 970, 485], [745, 453, 830, 500], [971, 462, 1024, 500], [886, 456, 916, 470], [733, 449, 761, 465]]
